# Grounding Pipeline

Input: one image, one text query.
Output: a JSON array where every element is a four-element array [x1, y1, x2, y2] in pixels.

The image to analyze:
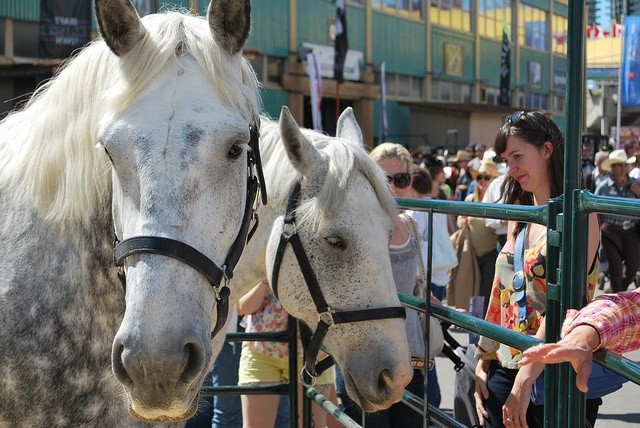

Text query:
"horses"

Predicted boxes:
[[227, 104, 415, 413], [0, 0, 263, 428]]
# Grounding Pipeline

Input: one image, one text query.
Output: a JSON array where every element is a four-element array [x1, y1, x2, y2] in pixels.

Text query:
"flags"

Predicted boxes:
[[38, 0, 92, 59], [333, 0, 349, 83], [498, 27, 512, 105], [619, 16, 640, 106]]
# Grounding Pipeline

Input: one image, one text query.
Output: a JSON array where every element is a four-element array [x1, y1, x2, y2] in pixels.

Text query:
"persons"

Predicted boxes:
[[595, 136, 617, 153], [335, 142, 441, 428], [591, 150, 610, 195], [624, 140, 640, 159], [473, 111, 601, 428], [185, 371, 214, 427], [327, 384, 345, 428], [211, 303, 292, 428], [402, 164, 459, 408], [628, 152, 640, 180], [234, 278, 336, 428], [401, 143, 502, 202], [580, 137, 596, 194], [517, 287, 640, 393], [594, 149, 640, 294]]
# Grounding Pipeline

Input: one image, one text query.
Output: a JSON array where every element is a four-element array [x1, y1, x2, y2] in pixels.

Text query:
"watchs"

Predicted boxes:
[[456, 162, 502, 320]]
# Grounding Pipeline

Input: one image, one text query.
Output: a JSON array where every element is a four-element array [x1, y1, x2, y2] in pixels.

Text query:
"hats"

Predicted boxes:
[[470, 160, 504, 179], [600, 149, 636, 171], [451, 150, 473, 162]]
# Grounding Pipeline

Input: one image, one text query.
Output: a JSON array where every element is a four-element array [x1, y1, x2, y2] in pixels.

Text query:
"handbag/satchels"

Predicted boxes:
[[397, 212, 444, 359], [512, 221, 630, 407]]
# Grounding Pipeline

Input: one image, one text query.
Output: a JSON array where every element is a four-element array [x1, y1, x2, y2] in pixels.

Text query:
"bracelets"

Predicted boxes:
[[262, 281, 269, 285]]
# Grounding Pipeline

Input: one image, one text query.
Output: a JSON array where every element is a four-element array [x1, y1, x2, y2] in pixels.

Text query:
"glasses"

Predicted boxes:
[[387, 173, 411, 188], [477, 175, 491, 180], [500, 111, 548, 134]]
